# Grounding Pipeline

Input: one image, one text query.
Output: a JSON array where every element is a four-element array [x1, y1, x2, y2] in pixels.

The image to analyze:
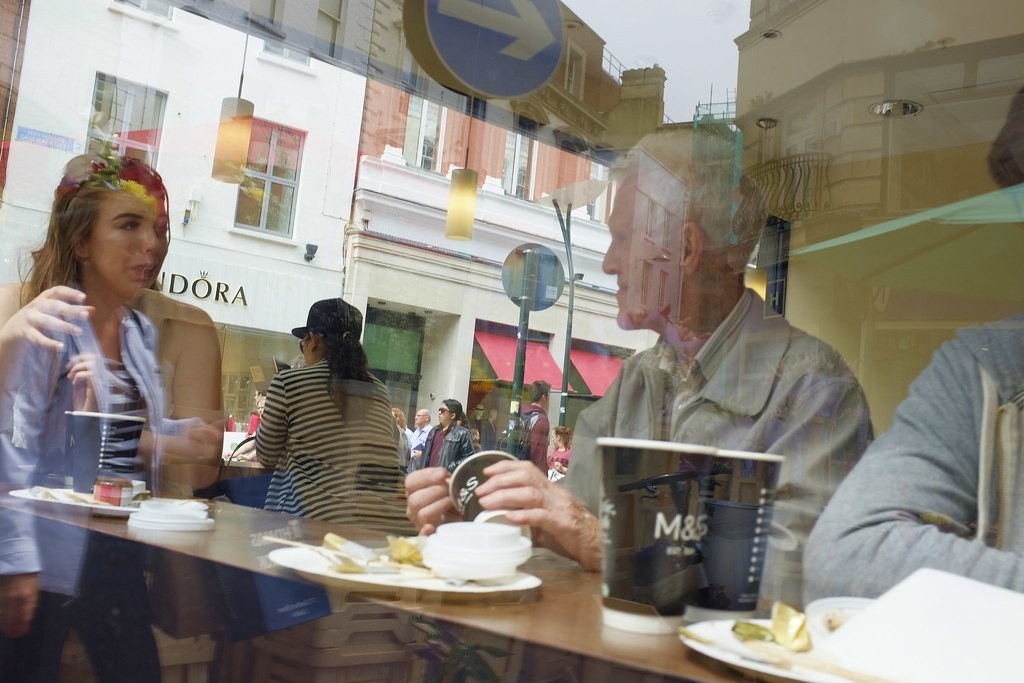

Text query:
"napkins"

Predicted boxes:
[[814, 566, 1024, 683]]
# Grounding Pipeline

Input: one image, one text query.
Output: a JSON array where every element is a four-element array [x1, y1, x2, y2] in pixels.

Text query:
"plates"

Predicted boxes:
[[267, 547, 541, 594], [681, 620, 848, 682], [9, 488, 137, 518]]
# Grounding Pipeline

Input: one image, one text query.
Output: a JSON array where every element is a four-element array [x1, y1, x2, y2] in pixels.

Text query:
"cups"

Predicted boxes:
[[75, 412, 144, 500], [595, 436, 713, 633], [682, 449, 786, 625], [805, 595, 883, 648], [448, 450, 533, 540], [64, 410, 80, 490]]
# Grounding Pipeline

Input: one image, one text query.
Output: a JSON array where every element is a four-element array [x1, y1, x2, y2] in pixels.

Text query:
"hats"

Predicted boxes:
[[292, 298, 362, 340]]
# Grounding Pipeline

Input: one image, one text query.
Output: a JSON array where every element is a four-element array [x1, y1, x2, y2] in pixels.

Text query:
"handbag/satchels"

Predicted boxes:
[[193, 468, 331, 642]]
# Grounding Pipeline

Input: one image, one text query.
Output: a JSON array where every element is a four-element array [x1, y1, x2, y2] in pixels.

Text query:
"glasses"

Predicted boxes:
[[439, 408, 454, 414], [55, 153, 163, 222], [299, 332, 324, 354], [476, 408, 484, 411]]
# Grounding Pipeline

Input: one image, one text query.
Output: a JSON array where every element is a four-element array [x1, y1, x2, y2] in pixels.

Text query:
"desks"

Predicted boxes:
[[0, 486, 772, 683]]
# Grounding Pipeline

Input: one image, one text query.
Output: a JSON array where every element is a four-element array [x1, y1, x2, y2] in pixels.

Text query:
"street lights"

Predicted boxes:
[[537, 177, 609, 426]]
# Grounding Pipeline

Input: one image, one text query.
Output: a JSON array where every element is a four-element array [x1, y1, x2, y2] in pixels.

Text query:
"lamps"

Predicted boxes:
[[212, 0, 255, 184], [443, 98, 478, 242], [305, 243, 318, 262]]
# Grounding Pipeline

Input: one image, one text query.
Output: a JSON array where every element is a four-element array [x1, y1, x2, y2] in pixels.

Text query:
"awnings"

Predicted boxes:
[[474, 331, 622, 397]]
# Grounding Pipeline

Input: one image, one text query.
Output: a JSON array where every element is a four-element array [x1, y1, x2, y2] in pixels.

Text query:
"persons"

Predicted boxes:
[[392, 399, 480, 473], [519, 380, 550, 479], [403, 126, 874, 611], [224, 390, 267, 462], [0, 264, 222, 683], [256, 298, 417, 537], [803, 86, 1024, 608], [0, 152, 223, 496], [548, 425, 573, 481], [468, 403, 497, 449]]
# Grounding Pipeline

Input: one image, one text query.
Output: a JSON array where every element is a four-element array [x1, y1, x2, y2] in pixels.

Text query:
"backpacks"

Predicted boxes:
[[495, 409, 548, 460]]
